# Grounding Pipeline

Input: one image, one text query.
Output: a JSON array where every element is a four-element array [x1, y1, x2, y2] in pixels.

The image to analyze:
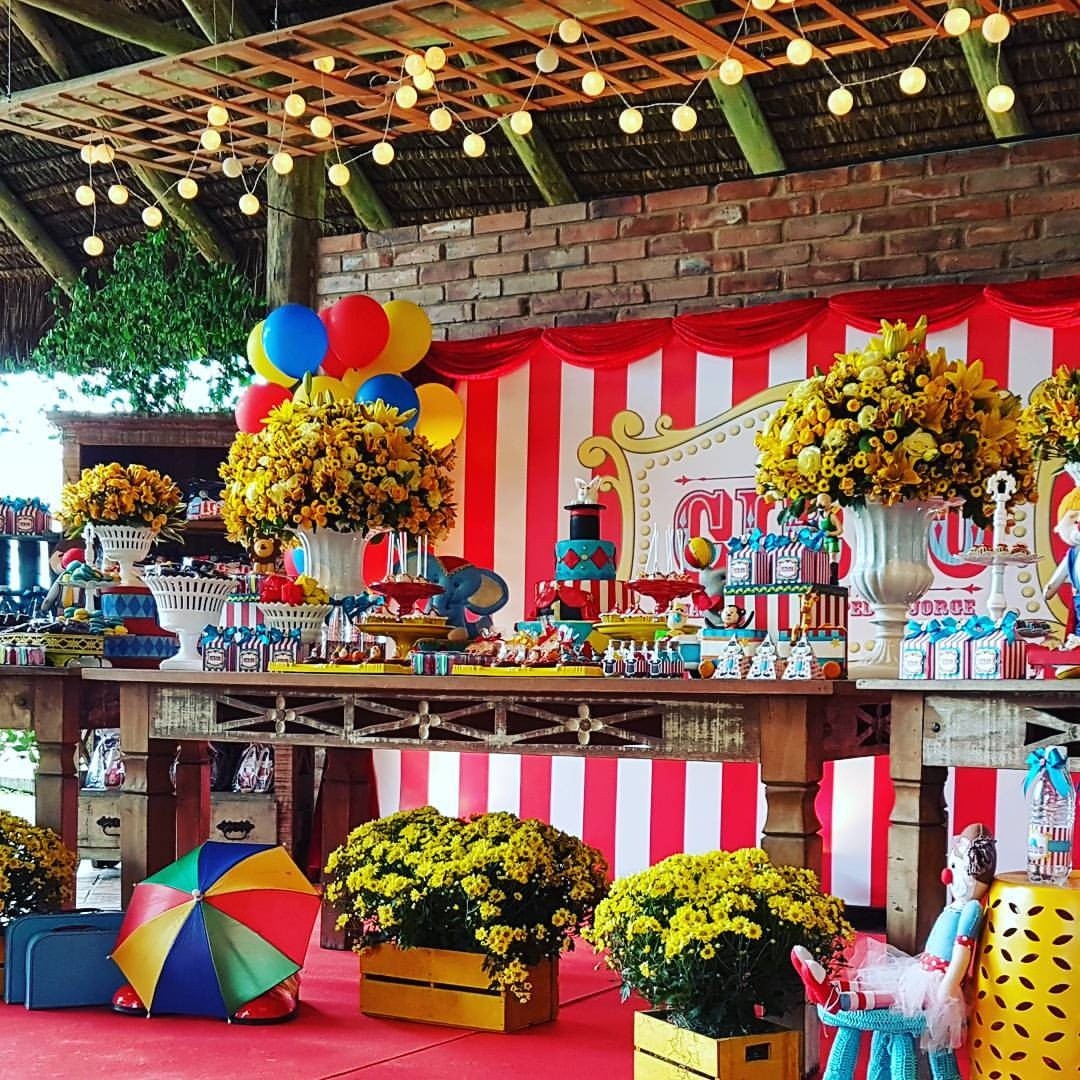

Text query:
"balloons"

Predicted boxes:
[[233, 293, 464, 453]]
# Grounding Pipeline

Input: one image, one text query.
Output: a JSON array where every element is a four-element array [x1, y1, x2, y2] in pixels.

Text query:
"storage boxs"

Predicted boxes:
[[929, 614, 979, 680], [200, 625, 237, 672], [771, 527, 830, 585], [898, 618, 940, 680], [269, 627, 304, 673], [236, 625, 270, 672], [727, 527, 776, 585], [969, 613, 1027, 680]]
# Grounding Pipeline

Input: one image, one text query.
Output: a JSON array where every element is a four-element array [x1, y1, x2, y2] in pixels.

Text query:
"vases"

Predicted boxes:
[[278, 521, 392, 598], [842, 496, 957, 681], [91, 525, 157, 584], [358, 942, 559, 1033], [632, 1008, 801, 1079]]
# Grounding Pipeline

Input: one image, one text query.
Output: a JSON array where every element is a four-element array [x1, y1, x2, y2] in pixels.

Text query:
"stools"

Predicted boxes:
[[817, 1004, 960, 1080]]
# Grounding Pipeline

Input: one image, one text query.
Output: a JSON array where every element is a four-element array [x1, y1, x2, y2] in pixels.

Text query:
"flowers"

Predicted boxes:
[[589, 849, 857, 1037], [753, 313, 1039, 530], [215, 371, 458, 544], [1017, 364, 1080, 465], [51, 462, 189, 547], [324, 805, 609, 1004], [0, 807, 82, 930]]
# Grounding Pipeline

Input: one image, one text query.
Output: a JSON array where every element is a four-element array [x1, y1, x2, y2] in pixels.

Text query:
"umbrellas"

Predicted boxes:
[[105, 837, 325, 1022]]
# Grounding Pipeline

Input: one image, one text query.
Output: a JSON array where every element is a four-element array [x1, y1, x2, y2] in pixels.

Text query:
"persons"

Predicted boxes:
[[789, 823, 998, 1053]]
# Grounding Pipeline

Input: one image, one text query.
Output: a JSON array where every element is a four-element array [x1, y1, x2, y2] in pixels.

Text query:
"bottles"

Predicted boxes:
[[29, 586, 49, 625], [0, 585, 19, 613], [1027, 745, 1076, 884]]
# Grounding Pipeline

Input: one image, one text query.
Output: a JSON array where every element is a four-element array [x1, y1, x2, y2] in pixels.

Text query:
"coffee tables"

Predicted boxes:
[[964, 867, 1080, 1079]]
